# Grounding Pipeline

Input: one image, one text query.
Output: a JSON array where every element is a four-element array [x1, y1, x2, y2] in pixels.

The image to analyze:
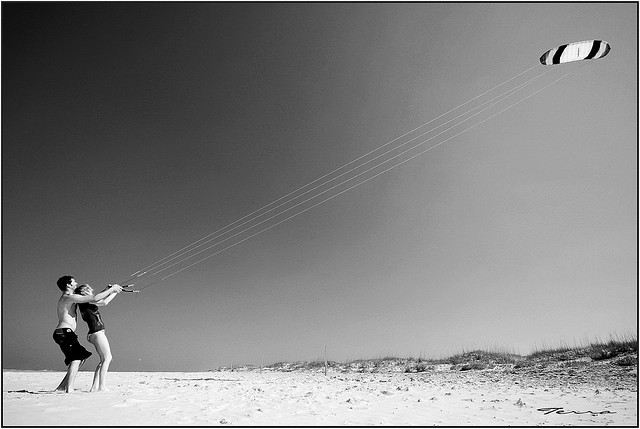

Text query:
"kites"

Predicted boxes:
[[539, 39, 611, 66]]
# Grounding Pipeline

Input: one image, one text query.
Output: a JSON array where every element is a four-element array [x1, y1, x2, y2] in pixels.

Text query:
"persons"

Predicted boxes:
[[53, 276, 122, 394], [74, 284, 122, 392]]
